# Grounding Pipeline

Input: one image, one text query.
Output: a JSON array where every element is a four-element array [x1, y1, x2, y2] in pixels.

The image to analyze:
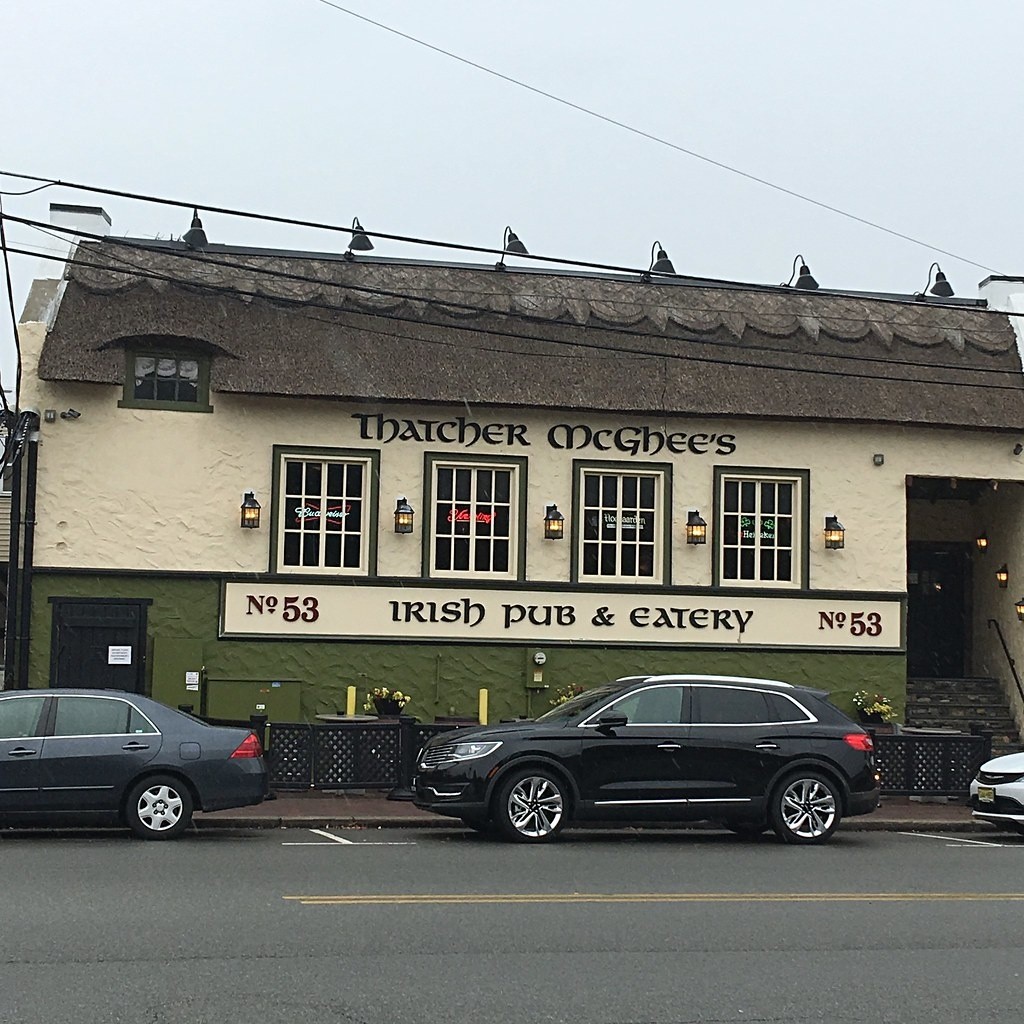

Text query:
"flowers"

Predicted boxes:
[[362, 685, 412, 713], [851, 689, 900, 722], [550, 682, 585, 708]]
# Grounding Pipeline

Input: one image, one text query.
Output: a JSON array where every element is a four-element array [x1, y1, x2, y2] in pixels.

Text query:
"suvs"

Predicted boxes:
[[407, 670, 882, 846]]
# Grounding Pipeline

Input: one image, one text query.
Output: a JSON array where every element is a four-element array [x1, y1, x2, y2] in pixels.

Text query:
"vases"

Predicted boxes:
[[858, 709, 882, 724], [373, 700, 405, 715]]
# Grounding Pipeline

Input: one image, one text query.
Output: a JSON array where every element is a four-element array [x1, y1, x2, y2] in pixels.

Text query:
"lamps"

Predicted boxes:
[[824, 515, 846, 549], [343, 217, 375, 261], [782, 254, 820, 291], [543, 504, 565, 539], [643, 240, 675, 283], [45, 410, 56, 421], [874, 454, 884, 466], [686, 510, 708, 545], [181, 208, 208, 252], [1014, 444, 1022, 455], [976, 534, 989, 553], [916, 262, 954, 302], [241, 492, 261, 529], [394, 497, 414, 534], [495, 226, 529, 271], [1015, 597, 1024, 621], [61, 408, 81, 418], [995, 563, 1008, 587]]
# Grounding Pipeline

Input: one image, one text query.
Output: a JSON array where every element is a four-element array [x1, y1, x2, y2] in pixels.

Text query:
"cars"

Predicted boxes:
[[968, 747, 1024, 836], [0, 687, 274, 841]]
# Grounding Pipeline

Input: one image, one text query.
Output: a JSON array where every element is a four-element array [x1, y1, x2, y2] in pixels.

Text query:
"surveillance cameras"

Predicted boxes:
[[1014, 445, 1023, 455], [68, 408, 81, 418]]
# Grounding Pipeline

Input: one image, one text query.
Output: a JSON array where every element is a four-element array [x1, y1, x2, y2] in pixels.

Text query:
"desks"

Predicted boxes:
[[315, 713, 378, 724], [901, 724, 961, 736]]
